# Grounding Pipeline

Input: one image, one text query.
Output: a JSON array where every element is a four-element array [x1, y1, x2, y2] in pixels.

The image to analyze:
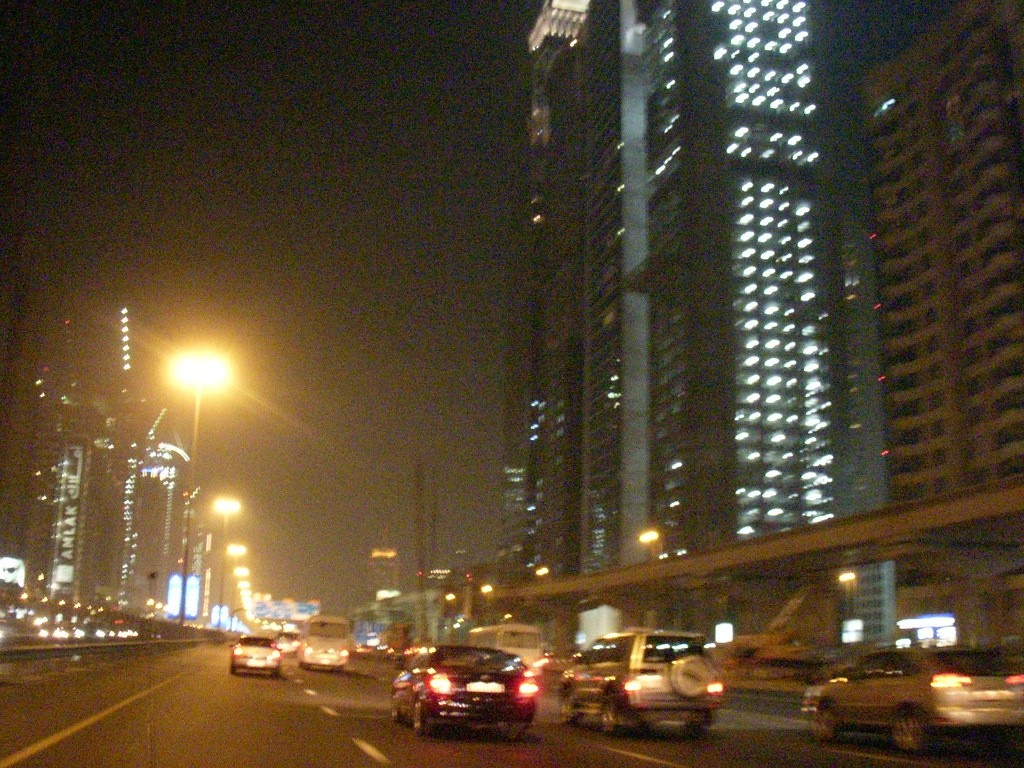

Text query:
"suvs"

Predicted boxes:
[[561, 627, 724, 740]]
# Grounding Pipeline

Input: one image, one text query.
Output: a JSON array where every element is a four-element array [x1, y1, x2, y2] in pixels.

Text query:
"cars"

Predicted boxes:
[[388, 641, 540, 743], [229, 634, 280, 677], [801, 642, 1024, 755]]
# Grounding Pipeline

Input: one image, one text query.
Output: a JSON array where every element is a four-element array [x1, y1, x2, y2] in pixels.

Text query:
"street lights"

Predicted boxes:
[[171, 346, 234, 637], [639, 530, 661, 623]]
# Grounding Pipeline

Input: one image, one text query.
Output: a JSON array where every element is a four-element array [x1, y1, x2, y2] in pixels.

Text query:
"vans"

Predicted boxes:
[[297, 614, 351, 673], [464, 620, 546, 687]]
